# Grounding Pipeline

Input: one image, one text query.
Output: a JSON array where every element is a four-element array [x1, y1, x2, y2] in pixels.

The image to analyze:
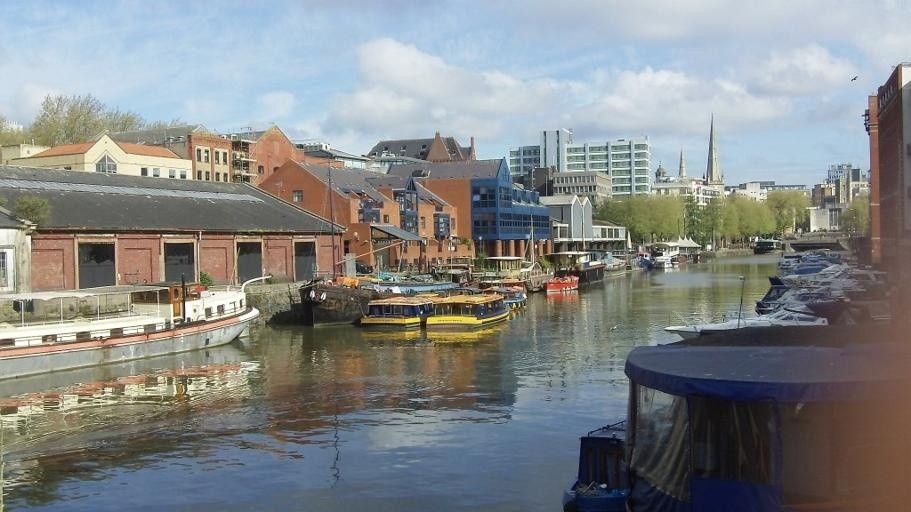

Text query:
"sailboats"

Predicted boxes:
[[298, 153, 381, 330]]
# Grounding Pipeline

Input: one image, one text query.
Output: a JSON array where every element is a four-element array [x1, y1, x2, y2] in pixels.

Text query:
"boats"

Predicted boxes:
[[754, 238, 891, 340], [666, 307, 833, 343], [563, 404, 672, 510], [542, 275, 579, 293], [601, 251, 627, 271], [544, 250, 608, 284], [359, 212, 555, 340], [0, 272, 259, 382]]
